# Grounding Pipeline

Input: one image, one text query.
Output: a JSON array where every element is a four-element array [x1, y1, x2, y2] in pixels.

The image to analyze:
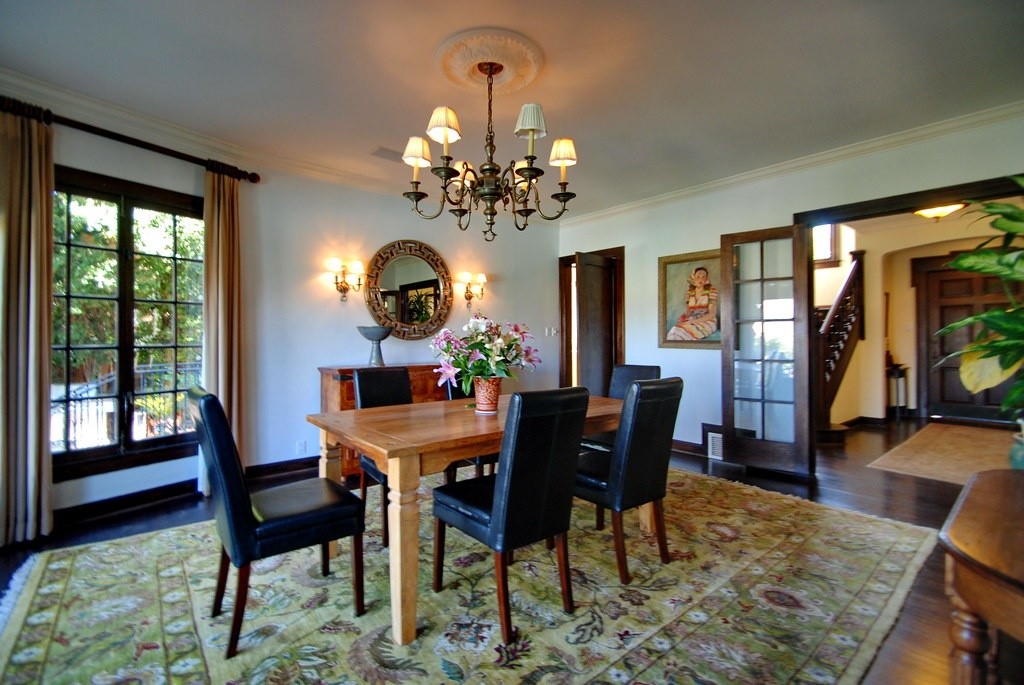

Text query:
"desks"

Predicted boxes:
[[307, 392, 657, 647], [317, 363, 502, 479], [938, 468, 1024, 685]]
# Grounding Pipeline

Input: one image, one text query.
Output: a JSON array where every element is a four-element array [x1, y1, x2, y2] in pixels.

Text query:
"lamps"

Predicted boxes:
[[453, 272, 487, 309], [402, 28, 576, 242], [320, 256, 365, 302]]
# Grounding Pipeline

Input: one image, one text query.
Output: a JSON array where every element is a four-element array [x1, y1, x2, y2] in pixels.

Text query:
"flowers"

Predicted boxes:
[[429, 309, 542, 396]]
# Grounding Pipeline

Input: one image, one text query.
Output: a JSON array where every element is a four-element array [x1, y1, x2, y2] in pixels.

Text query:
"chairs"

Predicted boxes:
[[545, 377, 684, 585], [443, 369, 499, 485], [353, 366, 413, 548], [188, 384, 365, 660], [579, 364, 661, 454], [432, 386, 589, 645]]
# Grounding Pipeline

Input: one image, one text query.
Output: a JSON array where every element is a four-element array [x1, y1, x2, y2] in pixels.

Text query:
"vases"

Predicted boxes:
[[472, 376, 504, 415]]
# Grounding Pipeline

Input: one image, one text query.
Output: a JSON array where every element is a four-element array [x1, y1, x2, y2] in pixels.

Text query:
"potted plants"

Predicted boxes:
[[931, 175, 1024, 470]]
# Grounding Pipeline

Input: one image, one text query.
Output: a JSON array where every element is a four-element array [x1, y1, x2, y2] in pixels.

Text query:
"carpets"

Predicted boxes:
[[865, 422, 1024, 485], [0, 442, 940, 685]]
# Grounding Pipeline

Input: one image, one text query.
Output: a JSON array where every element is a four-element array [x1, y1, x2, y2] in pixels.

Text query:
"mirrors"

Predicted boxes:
[[363, 239, 454, 341]]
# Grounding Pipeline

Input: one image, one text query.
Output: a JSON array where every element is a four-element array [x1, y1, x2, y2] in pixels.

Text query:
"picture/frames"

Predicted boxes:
[[657, 246, 741, 350]]
